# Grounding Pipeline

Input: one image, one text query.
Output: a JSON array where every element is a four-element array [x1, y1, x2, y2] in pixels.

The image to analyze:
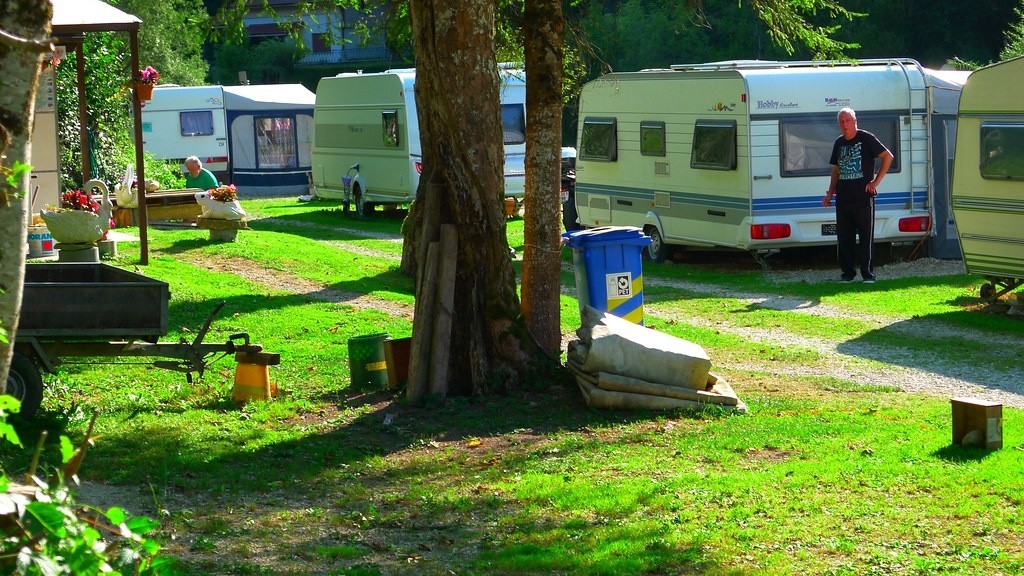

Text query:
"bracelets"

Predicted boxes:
[[826, 191, 836, 194]]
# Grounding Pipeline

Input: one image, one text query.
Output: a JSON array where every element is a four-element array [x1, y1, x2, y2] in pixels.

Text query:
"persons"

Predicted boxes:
[[823, 106, 895, 284], [185, 156, 219, 223]]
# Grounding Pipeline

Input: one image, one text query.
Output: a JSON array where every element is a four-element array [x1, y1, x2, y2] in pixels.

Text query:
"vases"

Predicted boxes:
[[137, 84, 153, 100]]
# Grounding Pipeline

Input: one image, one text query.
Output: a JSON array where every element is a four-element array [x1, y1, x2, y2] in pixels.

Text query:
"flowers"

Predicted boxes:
[[208, 182, 237, 203], [45, 57, 61, 68], [128, 65, 160, 85], [61, 189, 99, 213], [121, 172, 138, 189]]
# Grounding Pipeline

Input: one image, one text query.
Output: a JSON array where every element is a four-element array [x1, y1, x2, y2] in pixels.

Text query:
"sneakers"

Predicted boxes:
[[838, 275, 854, 284], [862, 277, 876, 284]]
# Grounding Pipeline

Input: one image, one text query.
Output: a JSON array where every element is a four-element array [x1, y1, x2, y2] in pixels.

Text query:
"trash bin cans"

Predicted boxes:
[[563, 225, 654, 327], [346, 332, 391, 389], [382, 337, 412, 390]]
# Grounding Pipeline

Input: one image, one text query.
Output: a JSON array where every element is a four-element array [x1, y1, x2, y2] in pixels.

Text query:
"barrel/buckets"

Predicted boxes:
[[26, 225, 58, 256], [348, 333, 390, 387], [232, 362, 272, 402]]
